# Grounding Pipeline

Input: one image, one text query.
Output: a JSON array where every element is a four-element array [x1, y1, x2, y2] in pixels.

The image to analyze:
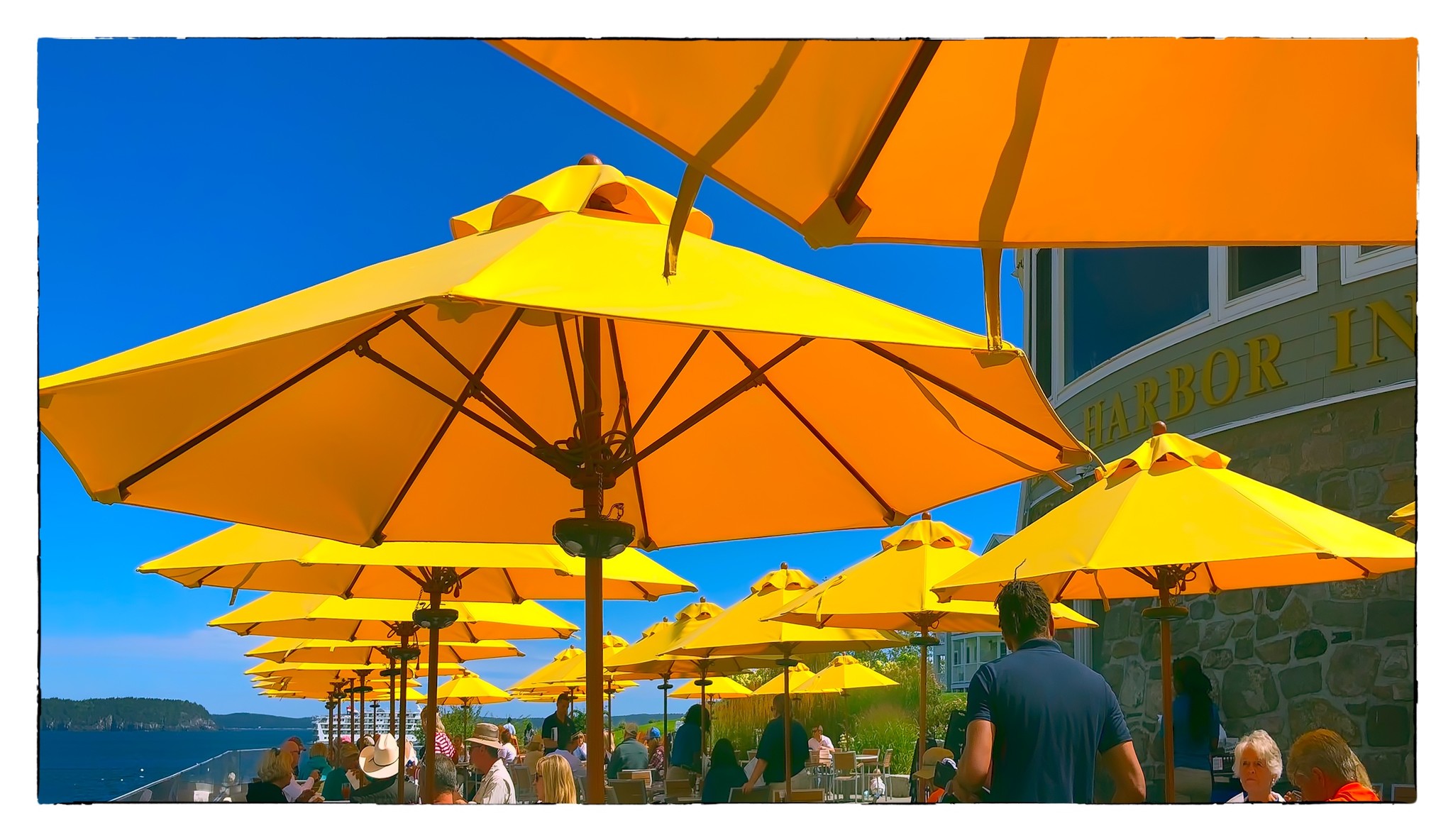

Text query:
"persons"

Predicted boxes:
[[1283, 728, 1381, 803], [666, 704, 710, 804], [642, 726, 670, 784], [870, 768, 886, 798], [1150, 656, 1226, 803], [607, 723, 649, 779], [246, 693, 615, 803], [1226, 729, 1286, 802], [948, 580, 1147, 804], [742, 693, 809, 802], [912, 747, 954, 803], [701, 738, 749, 804], [807, 725, 836, 796]]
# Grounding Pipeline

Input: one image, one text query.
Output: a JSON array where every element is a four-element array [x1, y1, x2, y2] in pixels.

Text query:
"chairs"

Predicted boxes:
[[468, 747, 894, 805], [139, 789, 152, 801]]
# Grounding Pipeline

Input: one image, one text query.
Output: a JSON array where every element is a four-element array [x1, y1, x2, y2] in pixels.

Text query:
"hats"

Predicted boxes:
[[358, 733, 411, 779], [464, 723, 505, 750], [911, 747, 954, 779], [288, 737, 307, 752], [625, 722, 638, 736], [342, 736, 351, 743], [641, 726, 661, 740], [340, 743, 359, 759], [531, 735, 545, 743], [361, 736, 375, 745]]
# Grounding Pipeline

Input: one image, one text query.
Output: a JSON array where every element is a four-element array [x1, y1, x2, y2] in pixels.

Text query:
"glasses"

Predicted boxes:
[[293, 750, 300, 755], [771, 706, 775, 712], [534, 772, 543, 783], [466, 746, 481, 751], [811, 730, 818, 734]]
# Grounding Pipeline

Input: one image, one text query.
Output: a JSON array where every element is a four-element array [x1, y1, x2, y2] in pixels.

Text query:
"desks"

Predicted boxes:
[[831, 754, 878, 803]]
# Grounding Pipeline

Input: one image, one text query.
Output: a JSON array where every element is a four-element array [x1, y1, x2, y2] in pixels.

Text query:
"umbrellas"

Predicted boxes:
[[243, 637, 527, 737], [207, 591, 581, 804], [136, 522, 697, 804], [478, 39, 1418, 350], [39, 153, 1104, 803], [243, 659, 514, 787], [504, 419, 1417, 804]]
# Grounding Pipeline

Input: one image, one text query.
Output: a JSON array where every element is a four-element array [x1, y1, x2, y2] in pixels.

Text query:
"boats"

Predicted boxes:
[[311, 703, 421, 745]]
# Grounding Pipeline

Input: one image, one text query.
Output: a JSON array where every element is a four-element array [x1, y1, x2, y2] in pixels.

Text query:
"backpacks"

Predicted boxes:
[[524, 729, 534, 743]]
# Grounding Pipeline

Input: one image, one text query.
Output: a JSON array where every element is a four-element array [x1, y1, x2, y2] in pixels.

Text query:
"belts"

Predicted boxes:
[[672, 764, 690, 770]]
